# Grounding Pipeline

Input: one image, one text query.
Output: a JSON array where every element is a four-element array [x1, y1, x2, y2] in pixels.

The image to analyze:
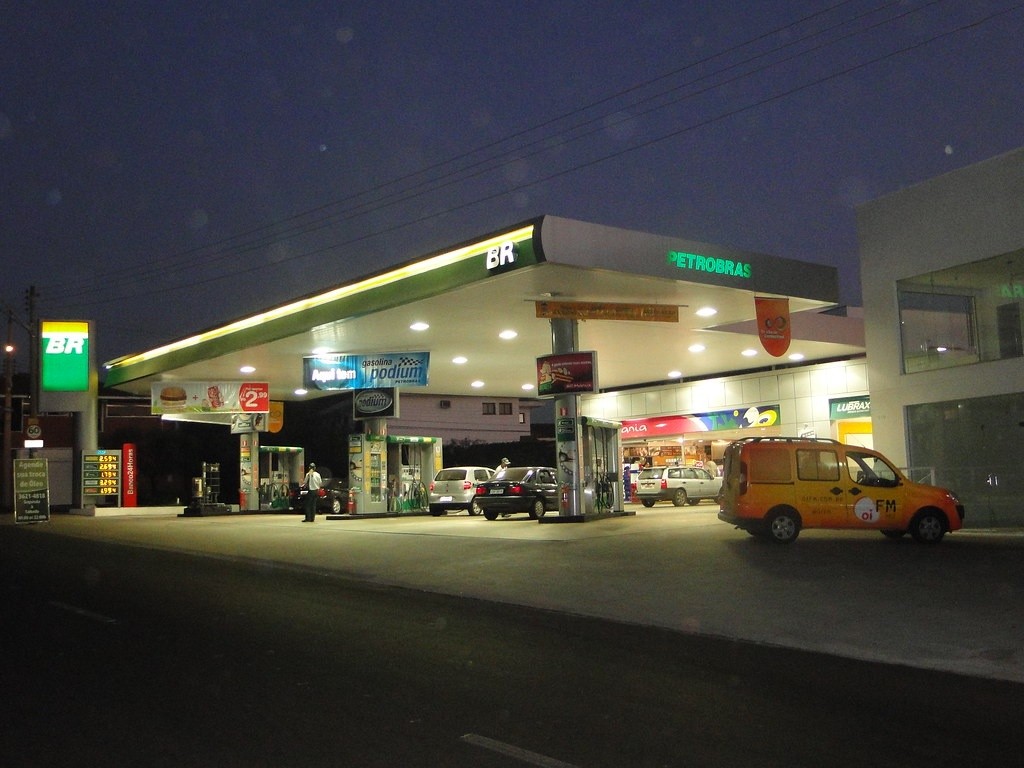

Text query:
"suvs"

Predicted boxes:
[[714, 436, 965, 544], [634, 465, 724, 507]]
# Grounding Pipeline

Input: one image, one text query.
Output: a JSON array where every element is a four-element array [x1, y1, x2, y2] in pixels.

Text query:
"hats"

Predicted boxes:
[[626, 465, 630, 469], [309, 463, 316, 467], [502, 457, 511, 465]]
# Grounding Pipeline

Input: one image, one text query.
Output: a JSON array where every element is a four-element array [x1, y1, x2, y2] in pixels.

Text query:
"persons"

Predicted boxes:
[[643, 462, 650, 469], [302, 462, 322, 522], [495, 457, 513, 517]]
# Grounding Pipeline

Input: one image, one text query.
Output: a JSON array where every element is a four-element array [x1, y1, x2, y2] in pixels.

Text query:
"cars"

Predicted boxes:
[[475, 466, 559, 520], [289, 478, 350, 514], [428, 466, 495, 516]]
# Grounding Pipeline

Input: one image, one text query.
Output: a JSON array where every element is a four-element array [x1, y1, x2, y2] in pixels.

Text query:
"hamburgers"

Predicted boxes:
[[160, 387, 187, 409]]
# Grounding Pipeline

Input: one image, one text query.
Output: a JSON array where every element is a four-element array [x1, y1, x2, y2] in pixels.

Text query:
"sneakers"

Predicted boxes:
[[302, 518, 315, 522]]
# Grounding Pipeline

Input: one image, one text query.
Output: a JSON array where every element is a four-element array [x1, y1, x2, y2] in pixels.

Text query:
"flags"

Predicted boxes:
[[754, 296, 792, 357]]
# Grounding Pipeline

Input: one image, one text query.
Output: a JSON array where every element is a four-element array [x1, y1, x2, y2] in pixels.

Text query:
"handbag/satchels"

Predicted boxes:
[[299, 484, 311, 501]]
